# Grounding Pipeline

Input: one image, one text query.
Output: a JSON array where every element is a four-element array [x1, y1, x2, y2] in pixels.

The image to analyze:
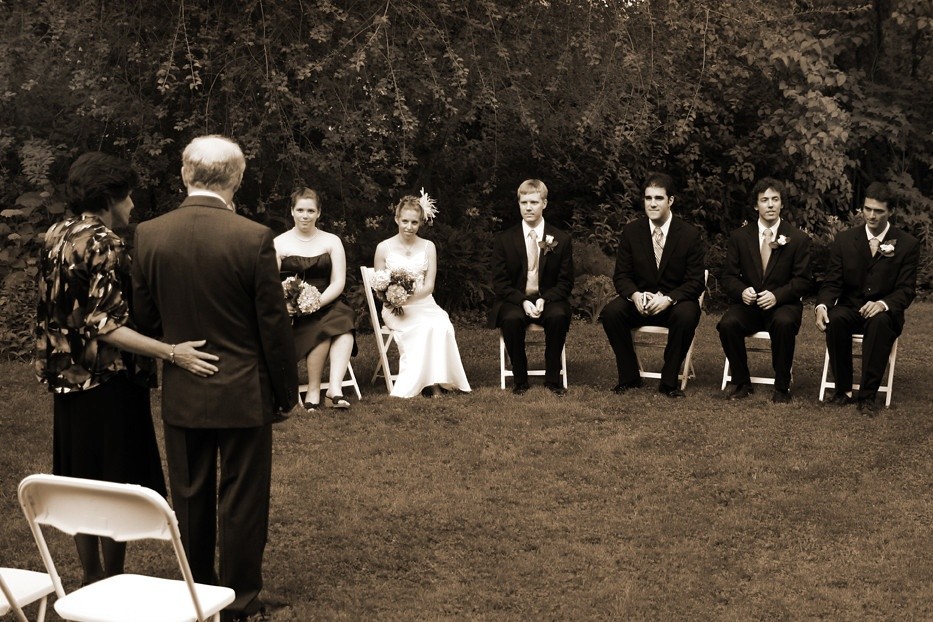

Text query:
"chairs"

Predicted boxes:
[[717, 330, 796, 393], [499, 319, 572, 392], [817, 332, 900, 411], [0, 472, 237, 622], [631, 268, 712, 392], [297, 265, 454, 405]]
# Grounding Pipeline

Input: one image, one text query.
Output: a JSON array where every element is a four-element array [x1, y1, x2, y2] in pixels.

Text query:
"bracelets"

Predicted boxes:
[[170, 344, 176, 365]]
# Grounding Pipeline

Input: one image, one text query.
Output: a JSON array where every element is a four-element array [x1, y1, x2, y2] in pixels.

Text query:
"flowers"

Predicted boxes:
[[875, 239, 896, 259], [769, 233, 792, 251], [281, 277, 322, 317], [419, 185, 440, 227], [538, 235, 559, 257], [367, 267, 419, 319]]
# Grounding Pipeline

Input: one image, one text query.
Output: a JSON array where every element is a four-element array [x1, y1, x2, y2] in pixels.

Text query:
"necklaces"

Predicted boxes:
[[399, 234, 415, 256], [293, 227, 318, 242]]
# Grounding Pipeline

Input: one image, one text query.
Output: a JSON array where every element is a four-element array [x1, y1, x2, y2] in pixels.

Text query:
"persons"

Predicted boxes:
[[599, 172, 706, 398], [716, 177, 811, 403], [487, 179, 574, 398], [132, 134, 298, 617], [31, 152, 219, 589], [374, 188, 472, 398], [273, 187, 359, 413], [815, 182, 919, 414]]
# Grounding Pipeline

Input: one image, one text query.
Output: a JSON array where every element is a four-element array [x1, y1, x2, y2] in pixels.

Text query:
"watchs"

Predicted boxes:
[[667, 296, 674, 305]]
